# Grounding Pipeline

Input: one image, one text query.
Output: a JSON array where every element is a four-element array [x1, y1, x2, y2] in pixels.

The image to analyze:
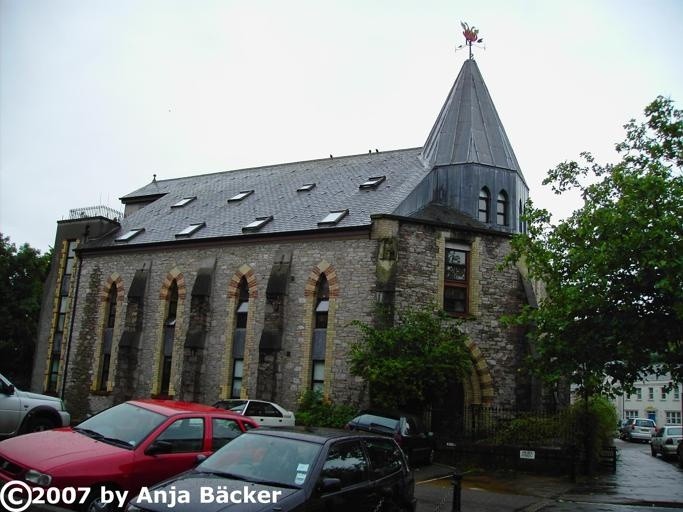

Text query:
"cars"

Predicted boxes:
[[649, 425, 683, 458], [0, 372, 71, 438], [0, 398, 437, 512]]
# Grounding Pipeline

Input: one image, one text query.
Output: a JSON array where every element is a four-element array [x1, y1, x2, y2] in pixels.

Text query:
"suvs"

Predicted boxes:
[[619, 417, 657, 443]]
